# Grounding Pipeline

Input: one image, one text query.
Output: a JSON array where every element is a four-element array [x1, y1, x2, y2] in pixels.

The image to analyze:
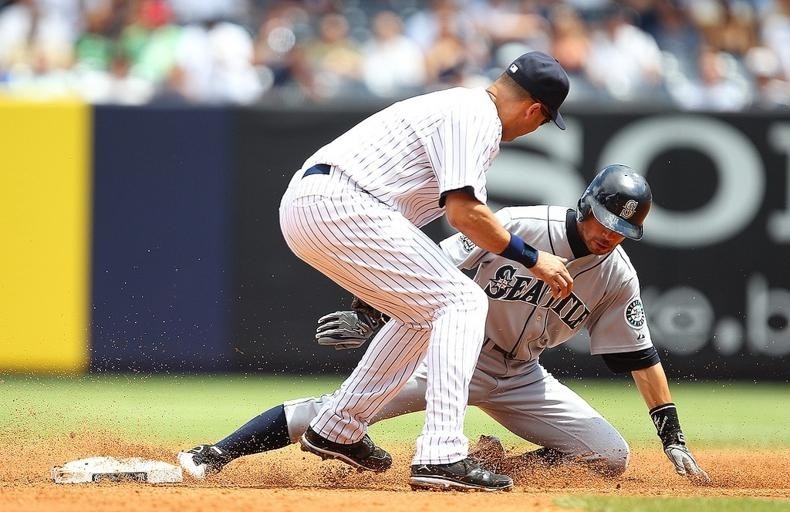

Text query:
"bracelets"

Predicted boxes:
[[496, 230, 538, 269]]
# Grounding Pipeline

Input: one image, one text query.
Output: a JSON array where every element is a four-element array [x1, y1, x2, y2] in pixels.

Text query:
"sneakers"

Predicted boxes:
[[409, 459, 513, 492], [299, 425, 392, 475], [179, 445, 232, 481]]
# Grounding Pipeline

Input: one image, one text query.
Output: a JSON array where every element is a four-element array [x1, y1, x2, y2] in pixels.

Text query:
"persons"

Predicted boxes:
[[0, 0, 790, 114], [173, 164, 713, 488], [279, 51, 575, 492]]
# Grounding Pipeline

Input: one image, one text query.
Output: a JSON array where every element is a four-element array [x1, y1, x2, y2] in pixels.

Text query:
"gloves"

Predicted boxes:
[[315, 311, 380, 350], [664, 444, 708, 476]]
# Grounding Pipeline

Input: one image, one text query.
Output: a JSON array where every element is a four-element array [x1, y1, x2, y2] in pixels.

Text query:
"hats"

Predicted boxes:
[[506, 50, 570, 130]]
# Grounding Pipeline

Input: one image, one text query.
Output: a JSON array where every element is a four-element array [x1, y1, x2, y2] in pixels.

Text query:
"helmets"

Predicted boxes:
[[575, 164, 653, 241]]
